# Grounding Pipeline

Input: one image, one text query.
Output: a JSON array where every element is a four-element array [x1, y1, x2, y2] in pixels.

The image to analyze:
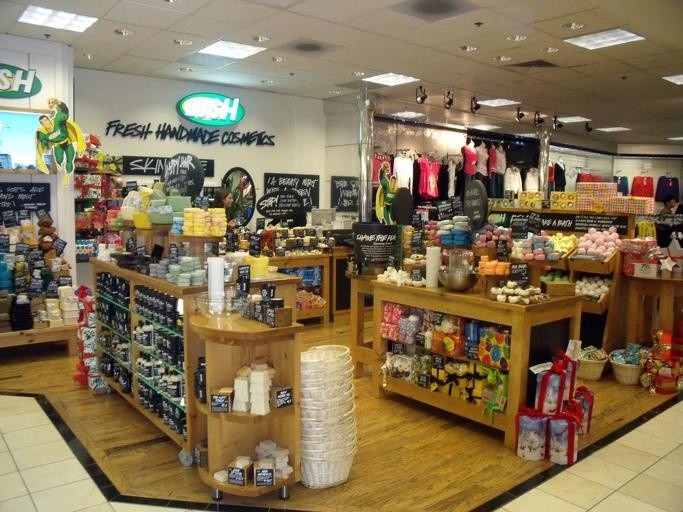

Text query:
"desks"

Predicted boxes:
[[369, 274, 588, 452], [330, 245, 374, 324], [266, 248, 332, 325], [345, 270, 377, 378], [618, 260, 683, 360]]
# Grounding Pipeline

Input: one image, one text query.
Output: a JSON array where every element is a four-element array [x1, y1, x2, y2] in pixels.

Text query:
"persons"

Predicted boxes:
[[658, 194, 675, 215], [379, 162, 398, 224], [36, 96, 74, 187], [214, 189, 237, 232]]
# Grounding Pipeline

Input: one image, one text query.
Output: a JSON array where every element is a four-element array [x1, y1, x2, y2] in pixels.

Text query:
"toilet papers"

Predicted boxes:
[[208, 257, 224, 310], [426, 244, 441, 288]]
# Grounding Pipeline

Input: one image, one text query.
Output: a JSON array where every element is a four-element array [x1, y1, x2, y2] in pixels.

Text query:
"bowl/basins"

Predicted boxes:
[[195, 293, 250, 320], [438, 269, 481, 292]]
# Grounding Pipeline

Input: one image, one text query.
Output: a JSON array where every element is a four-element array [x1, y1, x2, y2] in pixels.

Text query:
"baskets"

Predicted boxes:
[[608, 349, 643, 386], [299, 345, 357, 458], [576, 350, 609, 382], [299, 452, 359, 490]]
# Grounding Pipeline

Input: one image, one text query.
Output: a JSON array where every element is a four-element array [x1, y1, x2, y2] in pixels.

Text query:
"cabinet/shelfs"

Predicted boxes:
[[187, 312, 304, 500], [90, 221, 327, 466], [489, 207, 636, 375], [75, 168, 111, 249], [0, 270, 78, 357]]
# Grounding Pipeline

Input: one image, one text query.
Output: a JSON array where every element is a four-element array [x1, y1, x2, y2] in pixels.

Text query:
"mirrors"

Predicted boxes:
[[220, 166, 256, 229]]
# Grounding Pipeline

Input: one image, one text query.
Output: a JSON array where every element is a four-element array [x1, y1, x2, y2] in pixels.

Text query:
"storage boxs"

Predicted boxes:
[[537, 246, 577, 270], [567, 248, 617, 275]]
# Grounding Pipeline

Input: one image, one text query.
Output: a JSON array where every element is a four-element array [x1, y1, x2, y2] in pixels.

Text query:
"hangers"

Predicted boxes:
[[396, 149, 413, 160], [425, 151, 436, 160]]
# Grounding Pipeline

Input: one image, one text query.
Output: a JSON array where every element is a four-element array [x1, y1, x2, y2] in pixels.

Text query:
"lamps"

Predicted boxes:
[[584, 123, 592, 136], [553, 119, 563, 132], [533, 112, 544, 127], [416, 85, 427, 104], [514, 106, 525, 122], [445, 88, 453, 108], [471, 97, 480, 113]]
